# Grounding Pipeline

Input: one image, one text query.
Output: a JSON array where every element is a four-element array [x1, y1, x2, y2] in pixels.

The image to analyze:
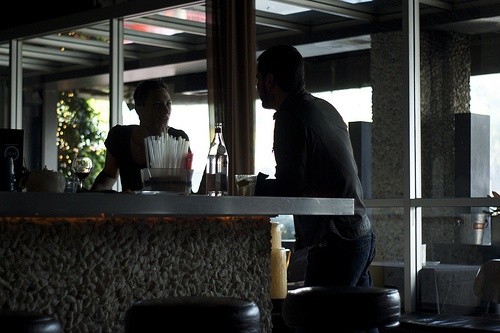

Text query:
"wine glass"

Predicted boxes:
[[72, 156, 92, 192]]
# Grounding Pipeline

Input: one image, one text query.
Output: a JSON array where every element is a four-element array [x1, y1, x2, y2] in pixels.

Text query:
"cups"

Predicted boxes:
[[140, 168, 194, 197], [234, 174, 257, 196]]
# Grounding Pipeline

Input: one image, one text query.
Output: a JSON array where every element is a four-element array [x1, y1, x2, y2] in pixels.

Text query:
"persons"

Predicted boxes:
[[235, 45, 376, 289], [89, 78, 189, 193]]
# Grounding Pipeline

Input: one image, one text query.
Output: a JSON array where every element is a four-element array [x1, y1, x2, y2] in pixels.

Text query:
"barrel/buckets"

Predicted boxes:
[[459, 212, 488, 244]]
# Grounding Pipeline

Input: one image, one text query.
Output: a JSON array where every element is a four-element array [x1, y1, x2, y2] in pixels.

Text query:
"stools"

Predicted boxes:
[[281, 285, 403, 333], [123, 292, 263, 333], [0, 307, 62, 333]]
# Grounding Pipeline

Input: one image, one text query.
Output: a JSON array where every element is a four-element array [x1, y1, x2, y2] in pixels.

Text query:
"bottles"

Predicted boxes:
[[205, 122, 229, 197], [270, 221, 282, 248], [269, 247, 287, 299]]
